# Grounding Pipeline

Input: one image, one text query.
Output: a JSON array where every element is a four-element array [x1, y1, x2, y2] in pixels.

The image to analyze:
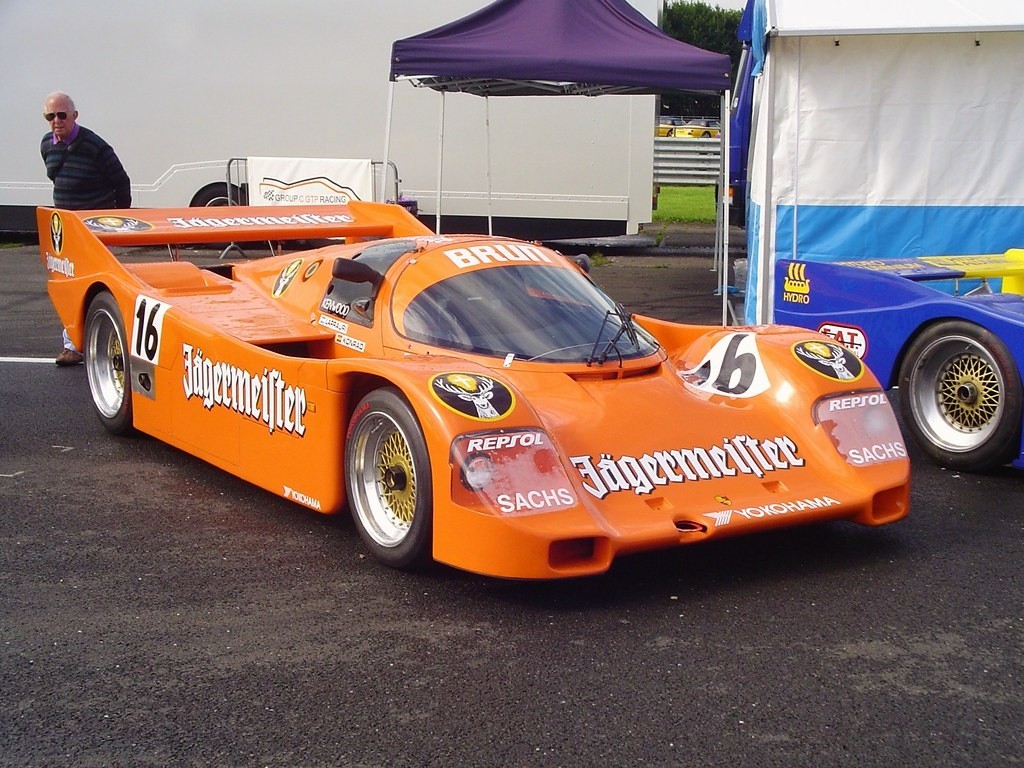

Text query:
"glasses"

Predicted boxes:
[[44, 112, 68, 121]]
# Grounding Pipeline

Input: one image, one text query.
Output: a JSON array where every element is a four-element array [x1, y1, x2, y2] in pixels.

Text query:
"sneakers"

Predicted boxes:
[[55, 349, 83, 366]]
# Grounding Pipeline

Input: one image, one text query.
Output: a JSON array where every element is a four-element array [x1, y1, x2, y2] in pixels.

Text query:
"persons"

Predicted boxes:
[[39, 91, 132, 367]]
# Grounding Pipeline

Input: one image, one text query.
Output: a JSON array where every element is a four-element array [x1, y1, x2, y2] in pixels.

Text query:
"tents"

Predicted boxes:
[[745, 0, 1024, 329], [379, 0, 734, 327]]
